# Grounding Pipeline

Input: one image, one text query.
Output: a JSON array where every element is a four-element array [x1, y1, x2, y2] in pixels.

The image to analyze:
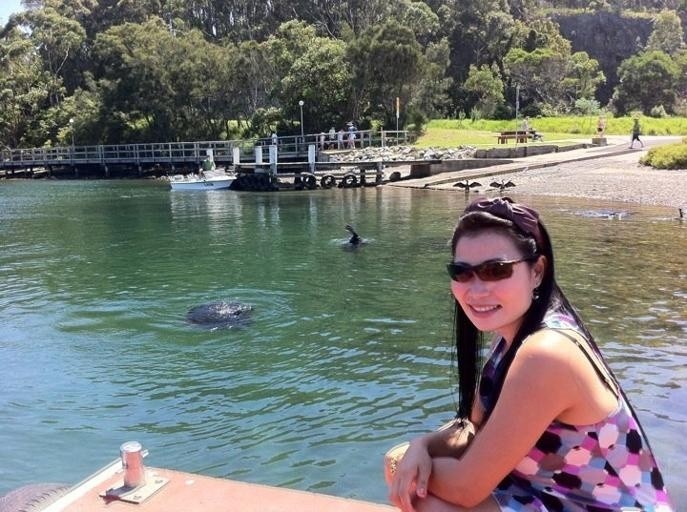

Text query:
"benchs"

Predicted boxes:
[[498, 131, 527, 144]]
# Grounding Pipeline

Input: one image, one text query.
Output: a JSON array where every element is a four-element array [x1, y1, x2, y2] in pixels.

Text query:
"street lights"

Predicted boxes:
[[298, 100, 305, 153], [68, 119, 74, 153]]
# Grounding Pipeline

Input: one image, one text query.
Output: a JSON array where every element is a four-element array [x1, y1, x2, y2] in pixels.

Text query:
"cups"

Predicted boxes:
[[120, 440, 149, 488]]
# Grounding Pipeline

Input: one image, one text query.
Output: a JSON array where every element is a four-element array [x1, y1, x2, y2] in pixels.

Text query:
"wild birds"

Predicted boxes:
[[453, 180, 482, 192], [490, 178, 516, 189]]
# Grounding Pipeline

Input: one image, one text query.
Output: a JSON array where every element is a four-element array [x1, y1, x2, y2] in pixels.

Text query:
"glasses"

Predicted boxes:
[[445, 256, 531, 283]]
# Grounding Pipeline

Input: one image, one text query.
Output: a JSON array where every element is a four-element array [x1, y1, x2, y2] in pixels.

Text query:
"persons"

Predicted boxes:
[[383, 195, 678, 512], [337, 129, 345, 150], [327, 126, 336, 149], [596, 116, 605, 138], [270, 131, 280, 151], [345, 122, 356, 150], [627, 118, 643, 149], [522, 116, 528, 132]]
